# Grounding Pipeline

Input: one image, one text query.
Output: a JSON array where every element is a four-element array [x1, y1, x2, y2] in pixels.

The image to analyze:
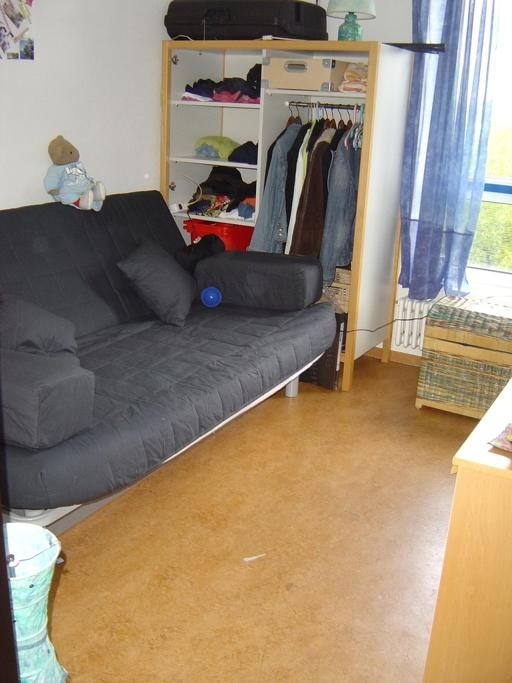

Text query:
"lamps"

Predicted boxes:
[[326, 0, 378, 42]]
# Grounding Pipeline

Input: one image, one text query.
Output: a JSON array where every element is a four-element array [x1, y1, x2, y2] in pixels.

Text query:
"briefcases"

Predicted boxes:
[[164, 0, 327, 39]]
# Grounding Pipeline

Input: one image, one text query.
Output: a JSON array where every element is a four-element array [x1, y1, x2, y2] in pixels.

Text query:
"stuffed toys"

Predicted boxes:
[[44, 134, 106, 212]]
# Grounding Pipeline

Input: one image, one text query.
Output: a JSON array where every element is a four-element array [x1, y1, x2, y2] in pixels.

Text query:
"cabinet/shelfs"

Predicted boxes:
[[158, 33, 416, 392]]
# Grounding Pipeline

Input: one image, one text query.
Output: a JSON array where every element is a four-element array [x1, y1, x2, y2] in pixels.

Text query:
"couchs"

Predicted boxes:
[[1, 187, 340, 537]]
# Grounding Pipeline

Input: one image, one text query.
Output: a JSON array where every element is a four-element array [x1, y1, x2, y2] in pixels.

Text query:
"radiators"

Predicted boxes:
[[394, 292, 434, 354]]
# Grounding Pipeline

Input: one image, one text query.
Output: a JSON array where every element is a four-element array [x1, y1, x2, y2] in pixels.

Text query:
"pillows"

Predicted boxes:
[[2, 235, 200, 355]]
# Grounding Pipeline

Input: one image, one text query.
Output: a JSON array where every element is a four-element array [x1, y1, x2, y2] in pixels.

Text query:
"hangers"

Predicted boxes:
[[268, 98, 364, 150]]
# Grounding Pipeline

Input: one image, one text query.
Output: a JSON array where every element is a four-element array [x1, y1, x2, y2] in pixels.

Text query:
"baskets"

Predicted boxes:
[[322, 267, 350, 311]]
